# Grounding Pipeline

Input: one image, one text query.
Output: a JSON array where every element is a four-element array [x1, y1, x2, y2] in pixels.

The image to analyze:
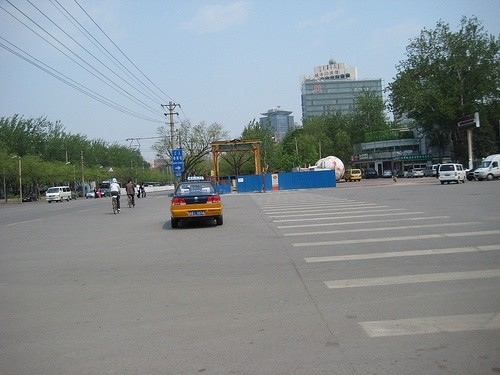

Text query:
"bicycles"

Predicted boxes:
[[127, 195, 135, 208], [111, 194, 121, 214]]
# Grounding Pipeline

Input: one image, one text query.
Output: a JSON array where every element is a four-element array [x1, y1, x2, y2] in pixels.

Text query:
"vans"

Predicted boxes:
[[344, 168, 362, 182], [424, 165, 438, 177], [45, 186, 71, 203], [474, 154, 500, 181], [438, 164, 466, 185]]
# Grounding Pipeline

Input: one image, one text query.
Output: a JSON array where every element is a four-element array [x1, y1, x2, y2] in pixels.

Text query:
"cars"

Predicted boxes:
[[466, 168, 477, 181], [85, 188, 110, 199], [167, 181, 224, 228], [70, 192, 79, 200], [406, 169, 424, 178], [22, 194, 39, 202], [383, 170, 392, 178]]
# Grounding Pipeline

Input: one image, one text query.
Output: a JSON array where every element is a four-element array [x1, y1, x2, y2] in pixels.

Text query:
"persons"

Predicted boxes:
[[392, 169, 398, 179], [125, 180, 135, 207], [110, 178, 121, 212], [136, 183, 146, 198]]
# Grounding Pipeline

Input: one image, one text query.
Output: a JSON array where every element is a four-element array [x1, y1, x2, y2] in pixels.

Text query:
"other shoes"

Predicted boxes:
[[117, 208, 120, 211]]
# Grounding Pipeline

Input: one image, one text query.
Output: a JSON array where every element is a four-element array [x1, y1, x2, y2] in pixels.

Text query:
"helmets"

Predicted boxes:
[[112, 178, 117, 183]]
